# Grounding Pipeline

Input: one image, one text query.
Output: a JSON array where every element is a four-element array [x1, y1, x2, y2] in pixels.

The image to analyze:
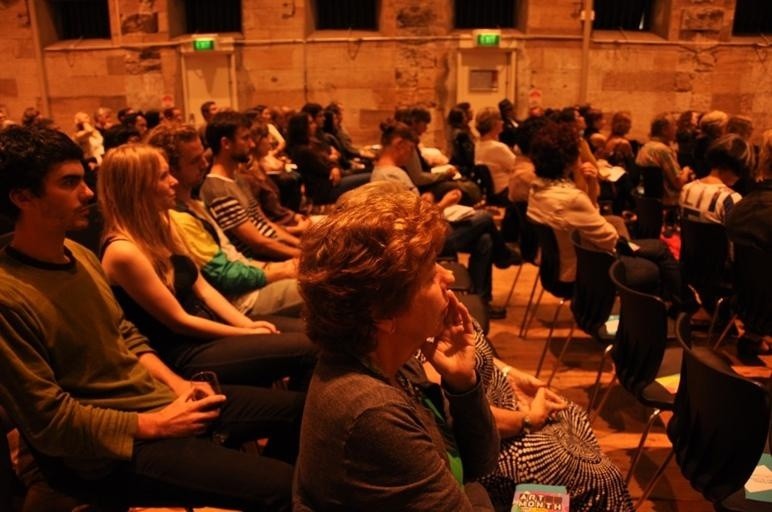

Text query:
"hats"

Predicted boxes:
[[499, 98, 514, 111]]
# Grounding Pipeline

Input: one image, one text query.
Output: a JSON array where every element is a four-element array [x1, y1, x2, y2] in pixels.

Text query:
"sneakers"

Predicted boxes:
[[488, 304, 506, 319], [497, 248, 522, 268], [738, 335, 771, 356]]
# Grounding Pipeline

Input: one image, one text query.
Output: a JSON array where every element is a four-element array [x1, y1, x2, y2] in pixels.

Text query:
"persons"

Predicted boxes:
[[366, 99, 520, 318], [507, 99, 771, 354], [419, 312, 636, 512], [293, 179, 520, 512], [2, 98, 373, 512]]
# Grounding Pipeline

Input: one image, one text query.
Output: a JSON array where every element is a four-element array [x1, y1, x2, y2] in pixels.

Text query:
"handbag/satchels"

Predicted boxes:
[[443, 204, 476, 222]]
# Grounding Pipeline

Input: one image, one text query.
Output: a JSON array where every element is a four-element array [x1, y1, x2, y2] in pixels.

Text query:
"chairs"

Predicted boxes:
[[600, 158, 771, 351], [591, 259, 733, 487], [455, 135, 576, 377], [548, 228, 678, 413], [634, 310, 772, 511]]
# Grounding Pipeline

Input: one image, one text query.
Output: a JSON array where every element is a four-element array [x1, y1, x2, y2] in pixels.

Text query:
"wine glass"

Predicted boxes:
[[190, 370, 226, 448]]
